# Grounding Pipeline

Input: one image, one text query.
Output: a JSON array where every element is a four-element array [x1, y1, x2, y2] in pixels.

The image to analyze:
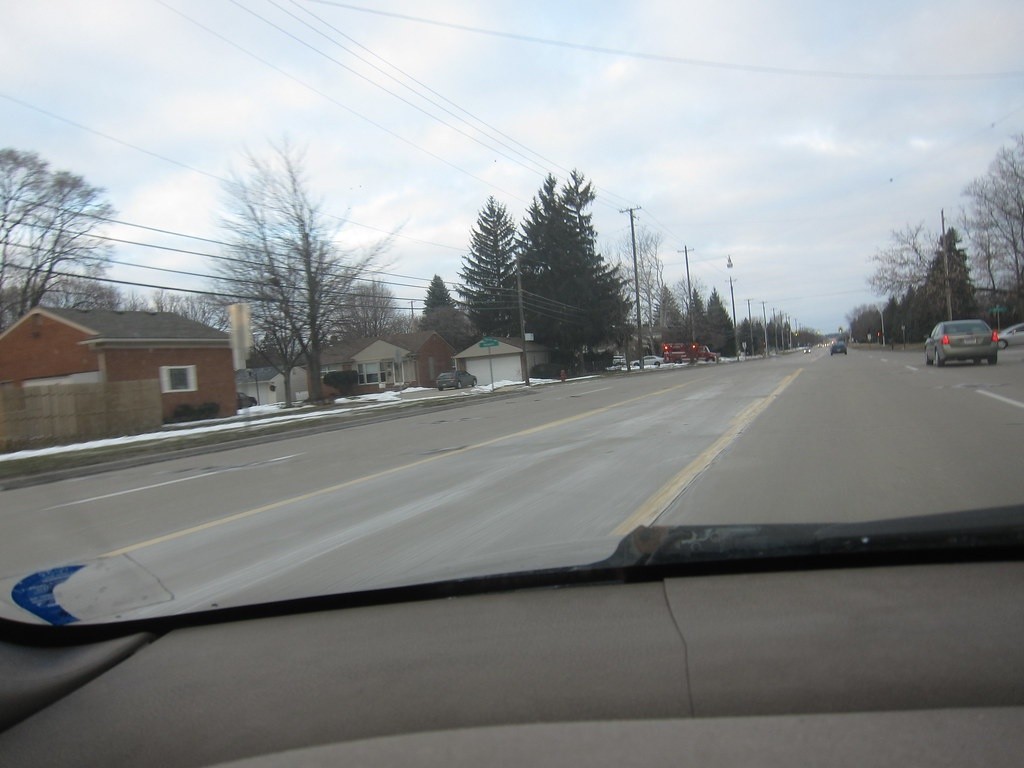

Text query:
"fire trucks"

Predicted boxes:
[[662, 343, 720, 365]]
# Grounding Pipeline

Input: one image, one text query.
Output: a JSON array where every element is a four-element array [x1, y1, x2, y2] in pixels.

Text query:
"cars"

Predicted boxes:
[[923, 318, 998, 367], [996, 323, 1024, 349], [435, 370, 478, 390], [829, 341, 848, 355], [630, 355, 664, 367], [612, 356, 626, 365], [803, 347, 811, 353], [236, 392, 257, 409]]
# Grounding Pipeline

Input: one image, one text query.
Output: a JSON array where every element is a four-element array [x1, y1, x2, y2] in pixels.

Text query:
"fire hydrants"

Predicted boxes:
[[560, 369, 566, 381]]
